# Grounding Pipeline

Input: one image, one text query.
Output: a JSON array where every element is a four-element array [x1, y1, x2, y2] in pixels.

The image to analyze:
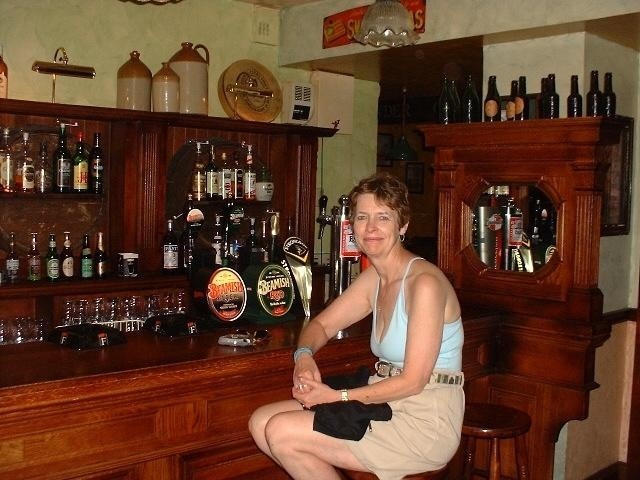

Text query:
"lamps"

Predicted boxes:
[[31, 45, 97, 103], [226, 72, 275, 119], [343, 0, 423, 48]]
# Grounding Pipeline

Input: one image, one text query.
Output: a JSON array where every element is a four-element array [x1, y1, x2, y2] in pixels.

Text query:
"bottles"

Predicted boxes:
[[0, 123, 103, 194], [472, 190, 524, 269], [5, 230, 105, 285], [161, 211, 280, 273], [190, 141, 256, 199], [528, 191, 556, 267], [0, 44, 9, 99], [438, 68, 616, 125], [167, 41, 210, 113], [150, 61, 182, 113], [116, 50, 153, 113]]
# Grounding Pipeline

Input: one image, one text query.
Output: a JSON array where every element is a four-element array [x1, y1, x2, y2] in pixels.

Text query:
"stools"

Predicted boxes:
[[345, 465, 448, 480], [459, 403, 533, 478]]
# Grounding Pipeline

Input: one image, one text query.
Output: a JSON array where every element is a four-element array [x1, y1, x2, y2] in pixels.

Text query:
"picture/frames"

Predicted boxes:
[[597, 114, 635, 237]]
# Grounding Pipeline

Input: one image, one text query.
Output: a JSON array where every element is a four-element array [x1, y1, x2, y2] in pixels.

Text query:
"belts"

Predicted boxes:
[[375, 361, 464, 384]]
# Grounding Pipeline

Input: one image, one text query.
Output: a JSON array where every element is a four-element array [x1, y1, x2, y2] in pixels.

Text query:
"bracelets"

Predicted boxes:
[[341, 389, 348, 402], [294, 347, 312, 362]]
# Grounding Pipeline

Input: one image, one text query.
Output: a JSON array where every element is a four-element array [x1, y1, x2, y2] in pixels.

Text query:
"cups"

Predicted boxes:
[[254, 183, 275, 201], [0, 290, 185, 346]]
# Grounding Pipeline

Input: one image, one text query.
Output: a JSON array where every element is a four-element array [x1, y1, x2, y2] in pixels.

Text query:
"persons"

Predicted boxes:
[[247, 173, 466, 480]]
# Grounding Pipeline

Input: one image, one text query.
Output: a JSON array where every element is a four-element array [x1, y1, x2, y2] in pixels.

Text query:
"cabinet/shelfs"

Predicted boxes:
[[0, 98, 339, 480]]
